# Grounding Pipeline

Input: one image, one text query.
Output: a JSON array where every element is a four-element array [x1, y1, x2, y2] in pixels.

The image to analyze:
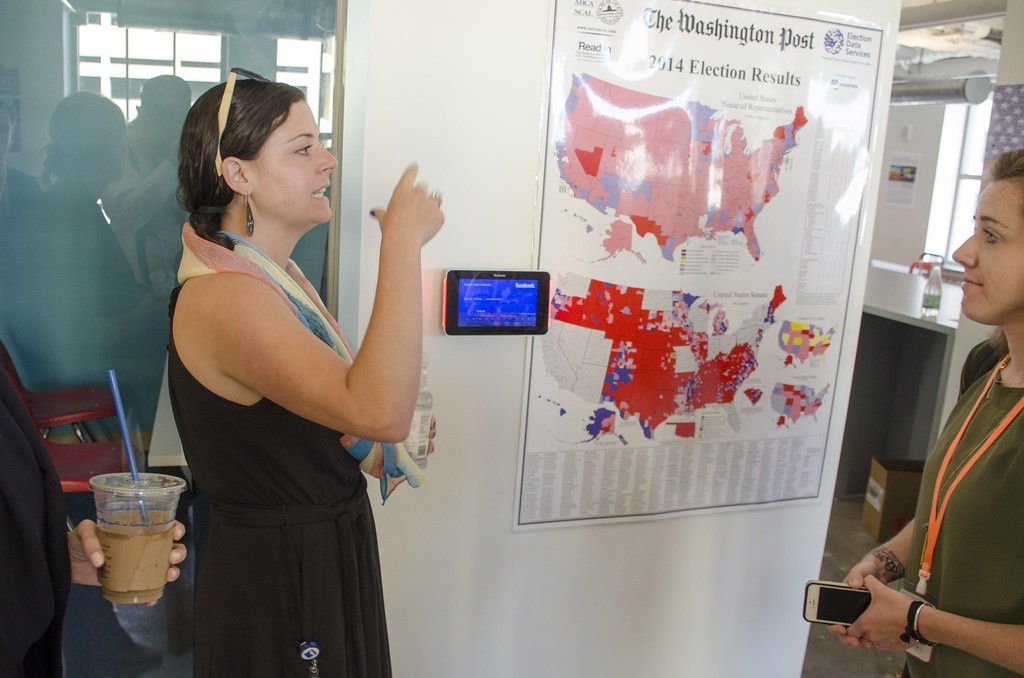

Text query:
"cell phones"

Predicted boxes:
[[802, 580, 872, 627]]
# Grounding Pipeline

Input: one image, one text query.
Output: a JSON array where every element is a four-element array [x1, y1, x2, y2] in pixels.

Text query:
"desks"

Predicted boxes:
[[832, 266, 965, 501], [148, 351, 193, 544]]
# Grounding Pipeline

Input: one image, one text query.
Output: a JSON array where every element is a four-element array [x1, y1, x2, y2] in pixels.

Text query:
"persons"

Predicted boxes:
[[165, 68, 445, 678], [827, 149, 1024, 678], [0, 73, 194, 442], [0, 368, 186, 678]]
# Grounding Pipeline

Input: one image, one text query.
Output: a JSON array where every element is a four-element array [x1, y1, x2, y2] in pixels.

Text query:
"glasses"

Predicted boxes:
[[209, 65, 267, 182]]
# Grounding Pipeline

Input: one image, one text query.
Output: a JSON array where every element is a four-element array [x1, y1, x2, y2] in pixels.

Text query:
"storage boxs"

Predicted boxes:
[[861, 456, 926, 543]]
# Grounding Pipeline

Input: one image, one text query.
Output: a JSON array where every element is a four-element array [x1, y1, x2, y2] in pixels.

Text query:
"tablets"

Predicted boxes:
[[444, 269, 550, 336]]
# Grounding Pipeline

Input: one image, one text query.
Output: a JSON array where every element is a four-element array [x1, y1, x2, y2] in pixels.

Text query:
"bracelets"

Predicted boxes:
[[898, 598, 937, 648]]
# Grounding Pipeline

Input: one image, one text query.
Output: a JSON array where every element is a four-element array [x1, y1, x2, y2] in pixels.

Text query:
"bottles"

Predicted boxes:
[[402, 353, 433, 471], [922, 260, 943, 317]]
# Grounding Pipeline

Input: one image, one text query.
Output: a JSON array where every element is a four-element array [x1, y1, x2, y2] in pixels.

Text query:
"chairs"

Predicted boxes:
[[0, 338, 129, 531]]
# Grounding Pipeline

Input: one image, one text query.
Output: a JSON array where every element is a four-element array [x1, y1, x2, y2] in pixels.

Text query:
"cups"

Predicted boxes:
[[88, 472, 188, 604]]
